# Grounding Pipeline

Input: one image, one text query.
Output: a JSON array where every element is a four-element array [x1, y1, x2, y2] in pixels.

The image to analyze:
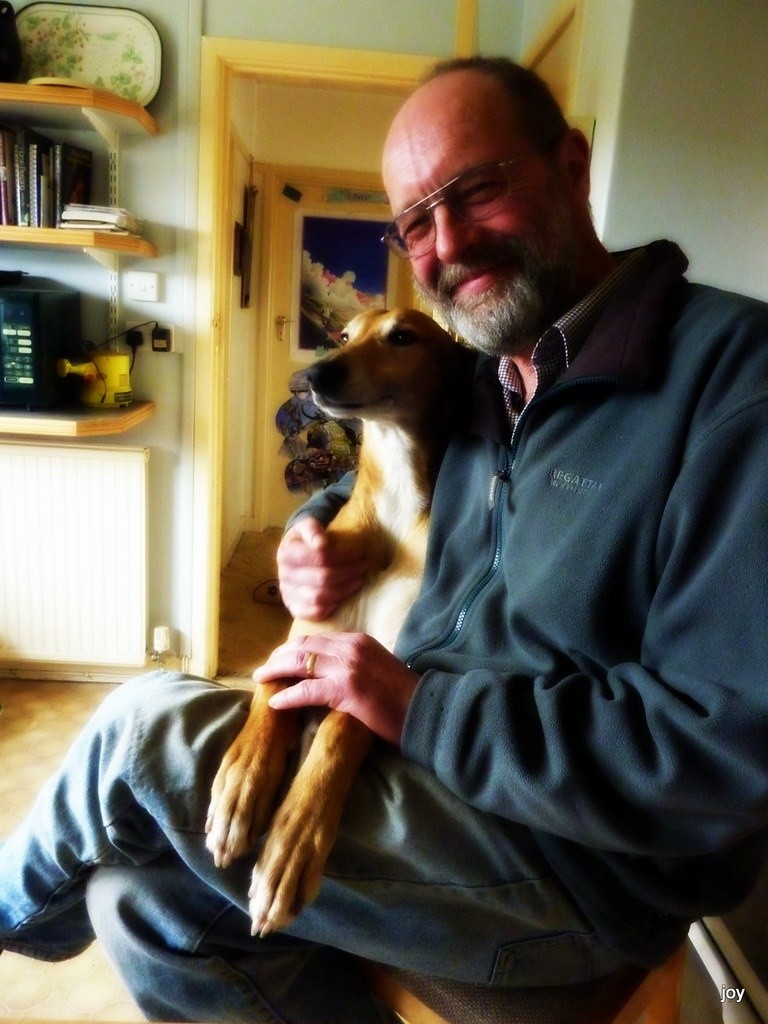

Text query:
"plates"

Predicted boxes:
[[14, 1, 163, 108]]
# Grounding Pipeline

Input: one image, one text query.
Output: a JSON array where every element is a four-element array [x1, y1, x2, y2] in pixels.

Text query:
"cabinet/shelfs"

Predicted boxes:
[[1, 82, 155, 439]]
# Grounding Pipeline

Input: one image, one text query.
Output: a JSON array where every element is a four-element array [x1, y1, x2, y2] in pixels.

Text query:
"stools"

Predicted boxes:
[[360, 932, 686, 1024]]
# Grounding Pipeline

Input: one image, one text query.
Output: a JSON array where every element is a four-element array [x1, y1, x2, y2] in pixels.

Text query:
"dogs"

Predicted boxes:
[[202, 306, 474, 943]]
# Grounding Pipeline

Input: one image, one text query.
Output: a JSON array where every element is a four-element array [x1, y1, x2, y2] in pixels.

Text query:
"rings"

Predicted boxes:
[[306, 652, 318, 679]]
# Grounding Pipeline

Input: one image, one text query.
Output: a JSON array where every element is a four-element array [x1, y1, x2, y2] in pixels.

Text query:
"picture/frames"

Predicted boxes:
[[289, 210, 396, 364]]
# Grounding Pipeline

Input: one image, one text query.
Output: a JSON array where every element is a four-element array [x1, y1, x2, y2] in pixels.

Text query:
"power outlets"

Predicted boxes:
[[123, 319, 177, 355]]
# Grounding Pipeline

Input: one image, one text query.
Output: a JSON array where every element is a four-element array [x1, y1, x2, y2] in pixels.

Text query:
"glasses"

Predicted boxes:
[[380, 143, 556, 260]]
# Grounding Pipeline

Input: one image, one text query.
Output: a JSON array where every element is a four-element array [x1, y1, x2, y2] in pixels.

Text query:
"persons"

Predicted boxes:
[[0, 55, 768, 1024]]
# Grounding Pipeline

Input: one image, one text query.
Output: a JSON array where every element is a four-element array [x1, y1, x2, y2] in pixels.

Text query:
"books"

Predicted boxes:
[[0, 127, 93, 229], [59, 202, 144, 240]]
